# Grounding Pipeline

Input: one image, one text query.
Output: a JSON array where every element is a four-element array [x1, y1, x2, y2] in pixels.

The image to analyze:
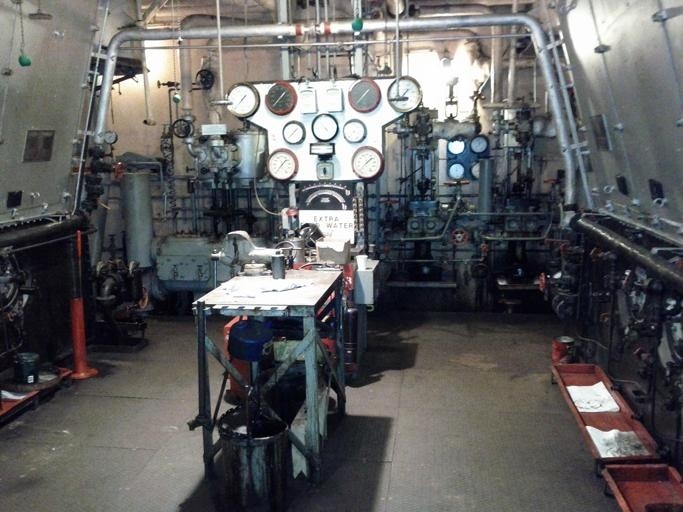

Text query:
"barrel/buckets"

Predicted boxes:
[[551, 335, 576, 384], [218, 408, 294, 512]]
